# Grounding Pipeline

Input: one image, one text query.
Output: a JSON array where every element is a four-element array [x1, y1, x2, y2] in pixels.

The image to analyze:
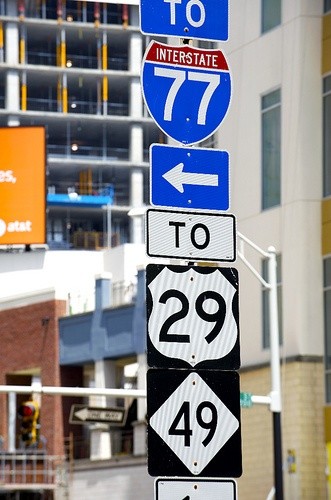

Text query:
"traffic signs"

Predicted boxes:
[[68, 404, 126, 429], [138, 0, 230, 43], [140, 40, 232, 146], [143, 209, 237, 263]]
